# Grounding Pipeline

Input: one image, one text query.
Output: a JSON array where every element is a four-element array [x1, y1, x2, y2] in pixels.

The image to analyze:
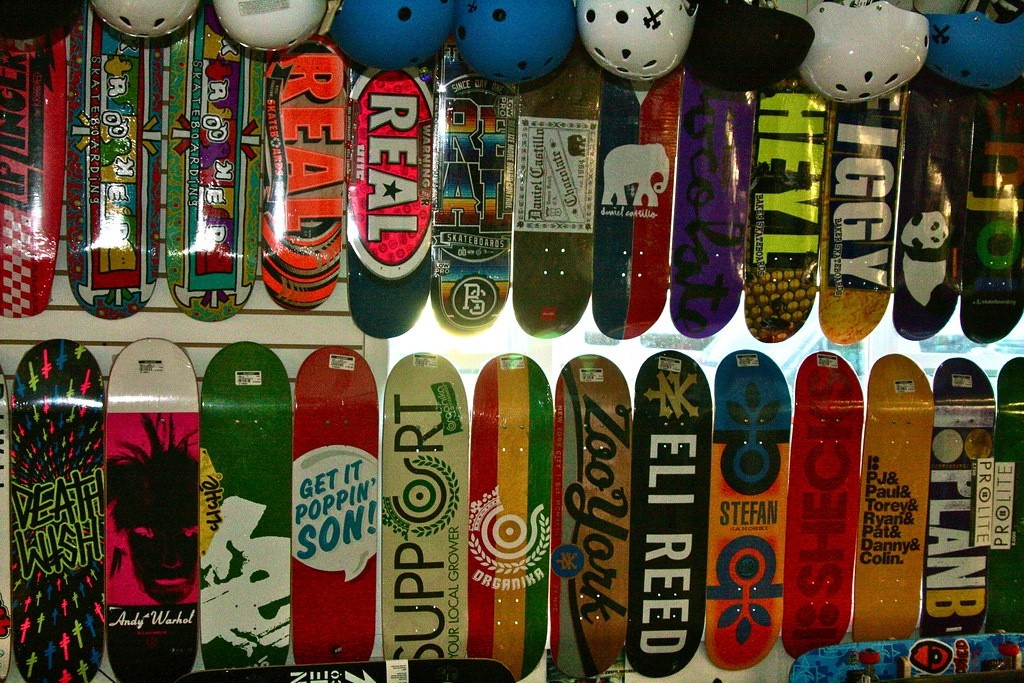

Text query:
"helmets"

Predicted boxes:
[[684, 0, 816, 92], [573, 0, 699, 81], [797, 1, 930, 103], [213, 0, 342, 54], [921, 11, 1024, 90], [90, 0, 201, 38], [455, 0, 577, 84], [328, 0, 454, 70]]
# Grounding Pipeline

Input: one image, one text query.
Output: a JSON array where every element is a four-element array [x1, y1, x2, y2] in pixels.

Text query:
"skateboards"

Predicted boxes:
[[2, 336, 1024, 683], [0, 0, 1024, 346]]
